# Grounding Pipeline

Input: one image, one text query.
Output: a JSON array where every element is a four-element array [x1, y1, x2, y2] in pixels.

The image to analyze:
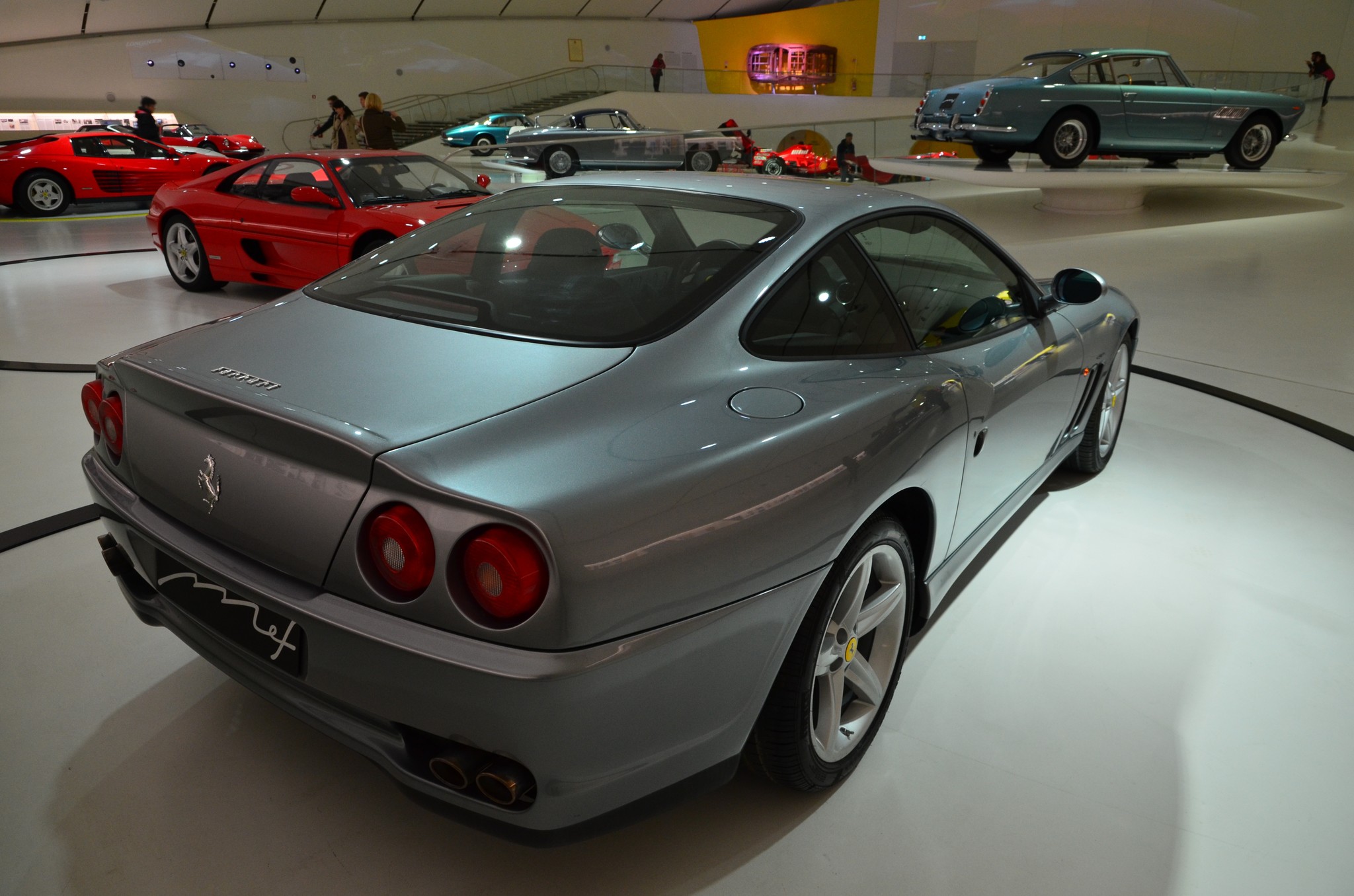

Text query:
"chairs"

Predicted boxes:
[[163, 130, 173, 137], [341, 166, 391, 202], [277, 172, 328, 208], [526, 228, 646, 334]]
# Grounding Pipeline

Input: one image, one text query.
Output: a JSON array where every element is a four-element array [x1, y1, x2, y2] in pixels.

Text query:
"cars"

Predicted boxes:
[[504, 107, 743, 177], [147, 148, 624, 298], [440, 112, 539, 156], [72, 123, 138, 138], [81, 164, 1143, 838], [0, 130, 247, 218], [911, 48, 1306, 172], [151, 123, 264, 160]]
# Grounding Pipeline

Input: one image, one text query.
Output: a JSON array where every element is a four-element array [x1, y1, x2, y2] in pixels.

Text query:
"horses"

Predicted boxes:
[[197, 454, 220, 515]]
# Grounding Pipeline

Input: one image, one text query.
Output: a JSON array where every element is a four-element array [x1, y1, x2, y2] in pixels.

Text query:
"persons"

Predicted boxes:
[[1306, 52, 1335, 106], [837, 133, 855, 182], [650, 53, 666, 92], [134, 95, 162, 155], [311, 91, 406, 151]]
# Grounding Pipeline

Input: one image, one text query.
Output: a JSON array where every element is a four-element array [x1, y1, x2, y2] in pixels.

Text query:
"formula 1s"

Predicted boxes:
[[719, 120, 892, 183]]
